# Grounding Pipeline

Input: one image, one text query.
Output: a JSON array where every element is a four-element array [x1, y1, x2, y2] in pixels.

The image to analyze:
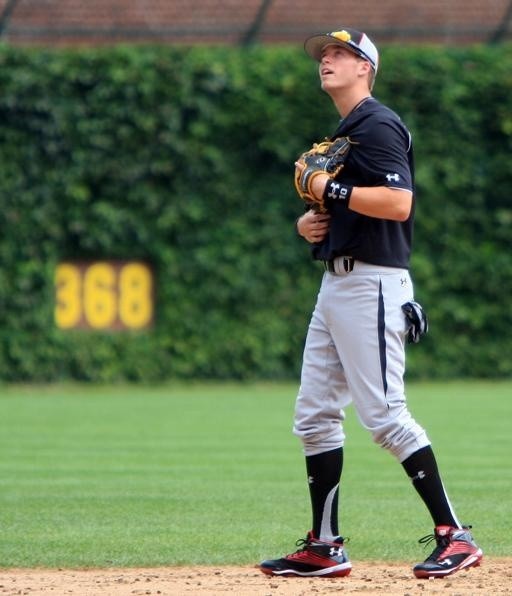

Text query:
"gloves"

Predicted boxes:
[[402, 301, 429, 345]]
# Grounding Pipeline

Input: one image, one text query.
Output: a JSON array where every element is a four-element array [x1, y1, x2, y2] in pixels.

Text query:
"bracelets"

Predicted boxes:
[[320, 178, 354, 210]]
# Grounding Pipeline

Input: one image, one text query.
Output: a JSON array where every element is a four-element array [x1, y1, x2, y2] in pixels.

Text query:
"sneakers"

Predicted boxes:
[[413, 526, 484, 578], [260, 531, 353, 576]]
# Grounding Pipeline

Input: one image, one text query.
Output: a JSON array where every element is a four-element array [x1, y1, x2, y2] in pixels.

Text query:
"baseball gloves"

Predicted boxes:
[[295, 137, 359, 215]]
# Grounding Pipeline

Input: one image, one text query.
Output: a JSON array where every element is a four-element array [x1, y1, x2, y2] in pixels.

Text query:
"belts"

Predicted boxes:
[[322, 258, 355, 273]]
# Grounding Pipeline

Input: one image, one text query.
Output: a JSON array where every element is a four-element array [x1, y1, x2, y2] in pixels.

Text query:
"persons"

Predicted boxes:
[[258, 27, 484, 583]]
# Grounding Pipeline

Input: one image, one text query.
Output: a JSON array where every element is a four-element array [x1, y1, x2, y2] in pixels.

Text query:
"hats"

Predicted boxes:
[[303, 26, 379, 74]]
